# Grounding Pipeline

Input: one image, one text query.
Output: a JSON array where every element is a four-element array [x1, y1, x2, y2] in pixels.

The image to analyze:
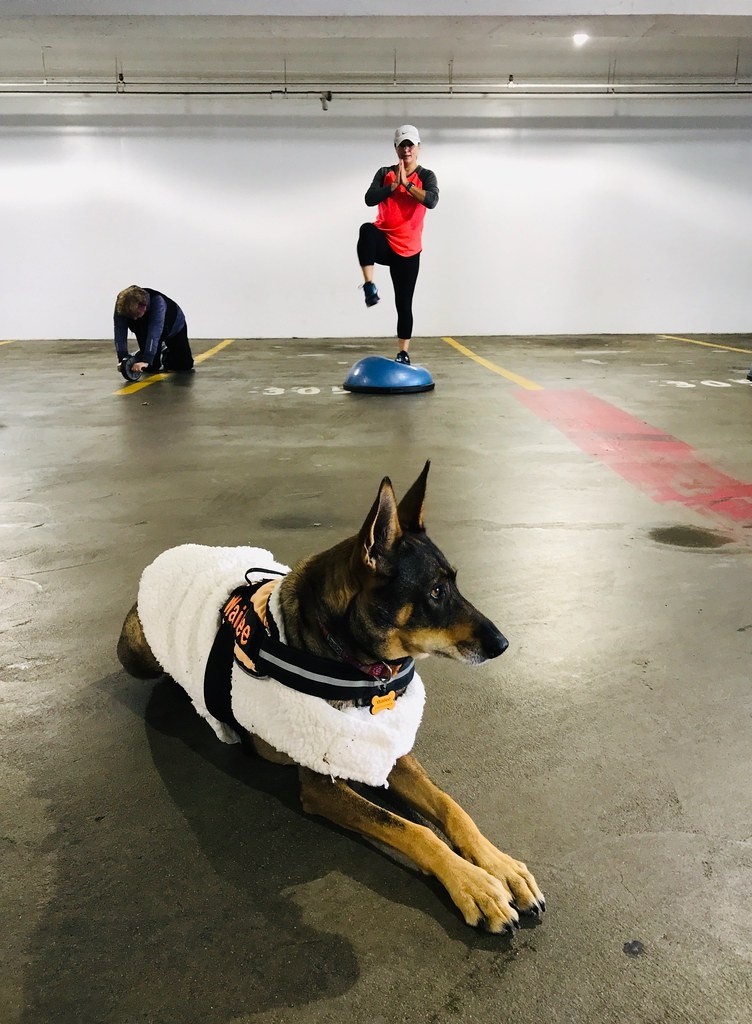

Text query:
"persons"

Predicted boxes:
[[111, 285, 196, 374], [356, 125, 439, 366]]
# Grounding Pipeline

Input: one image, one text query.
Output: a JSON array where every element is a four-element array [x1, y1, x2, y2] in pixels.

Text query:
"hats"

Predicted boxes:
[[394, 125, 420, 147]]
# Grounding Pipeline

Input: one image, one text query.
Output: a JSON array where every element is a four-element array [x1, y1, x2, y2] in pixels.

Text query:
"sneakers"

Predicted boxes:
[[362, 281, 380, 307], [395, 350, 410, 365]]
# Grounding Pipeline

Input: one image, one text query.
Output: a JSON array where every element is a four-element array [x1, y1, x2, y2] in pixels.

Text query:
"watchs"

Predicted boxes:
[[406, 181, 415, 191]]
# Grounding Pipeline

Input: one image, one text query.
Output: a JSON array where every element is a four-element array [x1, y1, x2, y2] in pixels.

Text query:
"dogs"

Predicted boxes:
[[116, 457, 545, 936]]
